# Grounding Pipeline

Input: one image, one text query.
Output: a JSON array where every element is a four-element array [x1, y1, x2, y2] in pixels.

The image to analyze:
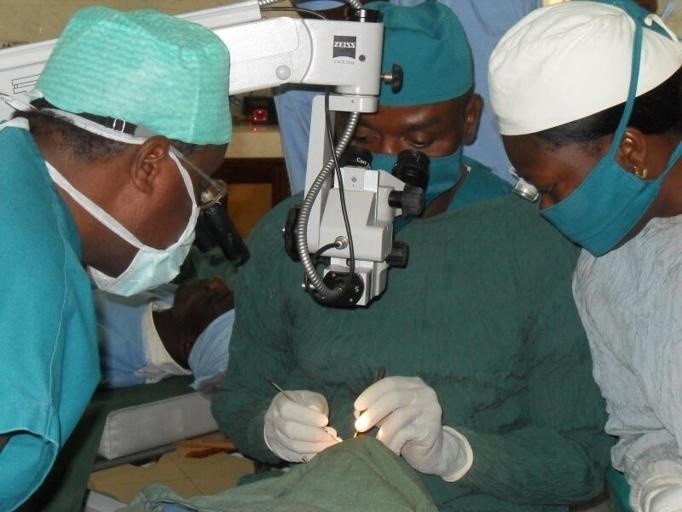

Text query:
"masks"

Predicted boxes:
[[368, 146, 462, 234], [537, 22, 682, 257], [27, 109, 200, 298]]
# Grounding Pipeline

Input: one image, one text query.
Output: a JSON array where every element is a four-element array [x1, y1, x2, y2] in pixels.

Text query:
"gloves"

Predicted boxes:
[[354, 375, 474, 483], [263, 391, 341, 465], [644, 485, 681, 511]]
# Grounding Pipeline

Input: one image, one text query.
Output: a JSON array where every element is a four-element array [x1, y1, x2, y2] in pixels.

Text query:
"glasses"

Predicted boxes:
[[169, 146, 227, 209]]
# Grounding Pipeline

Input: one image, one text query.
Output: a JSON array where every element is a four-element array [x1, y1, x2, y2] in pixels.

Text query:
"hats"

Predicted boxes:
[[488, 2, 681, 135], [363, 1, 474, 107], [32, 7, 232, 147], [189, 310, 235, 399]]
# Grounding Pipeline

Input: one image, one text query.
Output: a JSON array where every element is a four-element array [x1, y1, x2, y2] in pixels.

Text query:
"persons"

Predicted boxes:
[[487, 0, 681, 512], [211, 2, 617, 511], [0, 6, 234, 509], [85, 274, 236, 390]]
